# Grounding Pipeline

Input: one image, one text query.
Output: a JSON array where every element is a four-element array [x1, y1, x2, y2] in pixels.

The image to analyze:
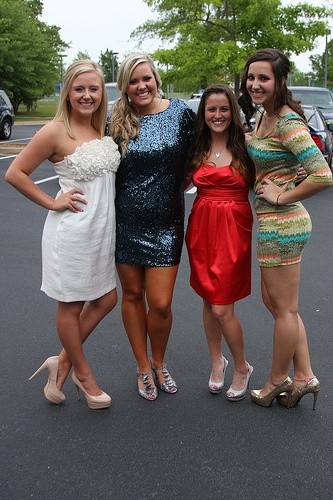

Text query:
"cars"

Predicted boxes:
[[102, 82, 122, 115], [185, 93, 202, 115], [243, 105, 333, 175]]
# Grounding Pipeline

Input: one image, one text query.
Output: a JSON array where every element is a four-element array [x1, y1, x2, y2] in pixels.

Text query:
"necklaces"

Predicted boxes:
[[212, 147, 227, 159]]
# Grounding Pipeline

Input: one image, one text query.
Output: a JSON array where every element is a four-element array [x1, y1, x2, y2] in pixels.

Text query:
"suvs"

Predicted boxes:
[[0, 88, 16, 141], [286, 86, 332, 134]]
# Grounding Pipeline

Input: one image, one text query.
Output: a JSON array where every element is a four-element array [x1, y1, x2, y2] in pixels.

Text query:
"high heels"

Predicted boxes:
[[276, 375, 321, 410], [250, 377, 293, 408], [209, 356, 229, 394], [72, 371, 111, 410], [29, 356, 66, 405], [226, 361, 254, 401]]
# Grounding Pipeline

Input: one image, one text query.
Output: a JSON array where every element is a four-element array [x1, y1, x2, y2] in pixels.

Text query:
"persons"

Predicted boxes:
[[5, 59, 121, 408], [184, 84, 307, 401], [238, 49, 333, 410], [104, 53, 199, 401]]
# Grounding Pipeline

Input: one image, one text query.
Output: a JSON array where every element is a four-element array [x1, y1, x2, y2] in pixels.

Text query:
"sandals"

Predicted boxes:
[[151, 360, 177, 394], [137, 367, 158, 401]]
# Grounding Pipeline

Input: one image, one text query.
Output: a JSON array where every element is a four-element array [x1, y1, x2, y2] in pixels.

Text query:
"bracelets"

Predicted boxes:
[[277, 193, 282, 206]]
